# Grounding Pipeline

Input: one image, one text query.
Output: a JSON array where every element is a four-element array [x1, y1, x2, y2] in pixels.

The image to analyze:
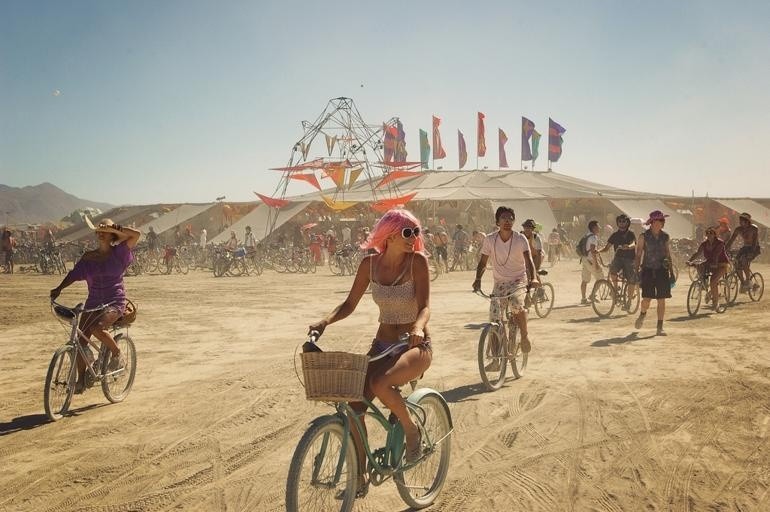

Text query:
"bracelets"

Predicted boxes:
[[112, 224, 122, 232]]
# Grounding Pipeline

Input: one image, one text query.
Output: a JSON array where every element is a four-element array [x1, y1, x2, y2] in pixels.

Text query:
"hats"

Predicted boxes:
[[617, 215, 630, 229], [520, 219, 536, 227], [84, 214, 129, 241], [737, 212, 751, 222], [716, 218, 729, 224], [643, 210, 671, 226]]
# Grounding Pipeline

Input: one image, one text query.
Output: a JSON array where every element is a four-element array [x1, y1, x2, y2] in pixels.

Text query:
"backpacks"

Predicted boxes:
[[575, 234, 594, 265]]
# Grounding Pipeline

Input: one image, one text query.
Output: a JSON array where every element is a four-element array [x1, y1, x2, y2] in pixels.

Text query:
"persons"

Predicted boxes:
[[50, 218, 140, 393], [576, 211, 760, 336], [145, 216, 566, 287], [472, 207, 540, 373], [307, 208, 430, 501], [1, 229, 16, 274], [43, 229, 55, 247]]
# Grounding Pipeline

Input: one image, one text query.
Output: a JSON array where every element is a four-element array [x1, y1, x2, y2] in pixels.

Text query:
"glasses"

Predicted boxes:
[[619, 220, 626, 223], [706, 231, 713, 234], [498, 215, 514, 222], [402, 226, 421, 240]]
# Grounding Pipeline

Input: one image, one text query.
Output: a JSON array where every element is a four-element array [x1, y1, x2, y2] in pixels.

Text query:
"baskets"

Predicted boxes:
[[112, 298, 136, 323], [298, 351, 369, 402]]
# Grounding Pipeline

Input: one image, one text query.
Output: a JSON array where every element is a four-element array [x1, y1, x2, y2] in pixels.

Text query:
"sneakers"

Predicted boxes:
[[521, 337, 531, 353], [581, 298, 592, 304], [610, 286, 621, 295], [588, 294, 601, 304], [334, 488, 367, 500], [635, 314, 646, 329], [740, 284, 746, 294], [109, 349, 124, 371], [484, 361, 500, 372], [743, 279, 752, 289], [656, 329, 668, 336], [66, 383, 83, 395], [621, 303, 630, 311], [406, 421, 423, 464]]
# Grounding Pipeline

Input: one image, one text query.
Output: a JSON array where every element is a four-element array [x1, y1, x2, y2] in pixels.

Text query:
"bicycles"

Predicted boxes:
[[689, 256, 733, 282], [548, 244, 559, 267], [725, 252, 764, 303], [44, 298, 136, 422], [684, 259, 730, 316], [671, 261, 678, 284], [524, 270, 554, 319], [590, 249, 641, 318], [285, 329, 454, 512], [473, 280, 541, 391]]
